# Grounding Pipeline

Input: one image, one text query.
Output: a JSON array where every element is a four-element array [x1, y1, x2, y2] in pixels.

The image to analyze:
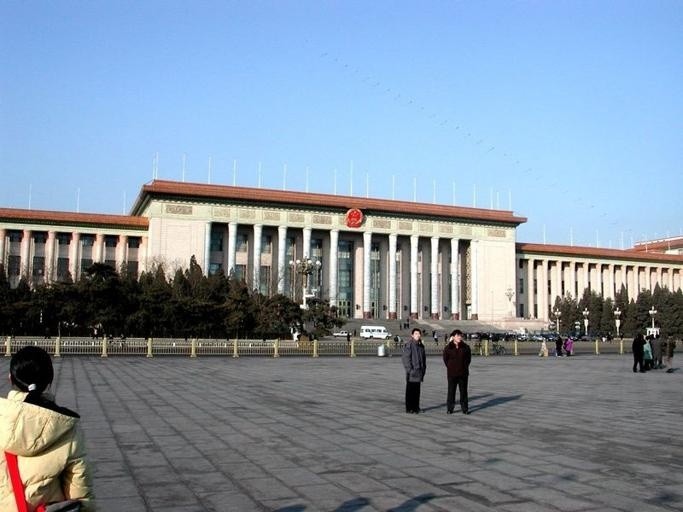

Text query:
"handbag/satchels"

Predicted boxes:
[[36, 499, 80, 511]]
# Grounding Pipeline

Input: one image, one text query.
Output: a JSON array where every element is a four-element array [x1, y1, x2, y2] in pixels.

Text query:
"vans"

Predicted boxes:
[[359, 325, 392, 340]]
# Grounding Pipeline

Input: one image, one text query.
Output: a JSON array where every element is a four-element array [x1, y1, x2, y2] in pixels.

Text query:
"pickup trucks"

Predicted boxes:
[[332, 331, 352, 337]]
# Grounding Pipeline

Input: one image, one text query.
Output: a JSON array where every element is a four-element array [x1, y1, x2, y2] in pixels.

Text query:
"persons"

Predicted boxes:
[[442, 328, 471, 415], [632, 330, 676, 373], [498, 337, 506, 356], [92, 327, 98, 339], [402, 326, 426, 414], [391, 316, 447, 345], [541, 335, 574, 357], [0, 341, 94, 511], [109, 334, 114, 344], [120, 334, 126, 341]]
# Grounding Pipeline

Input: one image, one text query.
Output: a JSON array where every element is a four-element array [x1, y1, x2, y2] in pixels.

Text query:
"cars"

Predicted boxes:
[[462, 330, 615, 342]]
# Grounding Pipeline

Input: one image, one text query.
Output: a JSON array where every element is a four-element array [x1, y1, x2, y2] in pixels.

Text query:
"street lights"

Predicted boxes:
[[288, 256, 321, 310], [505, 288, 514, 316], [582, 307, 590, 335], [649, 306, 657, 336], [553, 307, 562, 334], [613, 306, 621, 336]]
